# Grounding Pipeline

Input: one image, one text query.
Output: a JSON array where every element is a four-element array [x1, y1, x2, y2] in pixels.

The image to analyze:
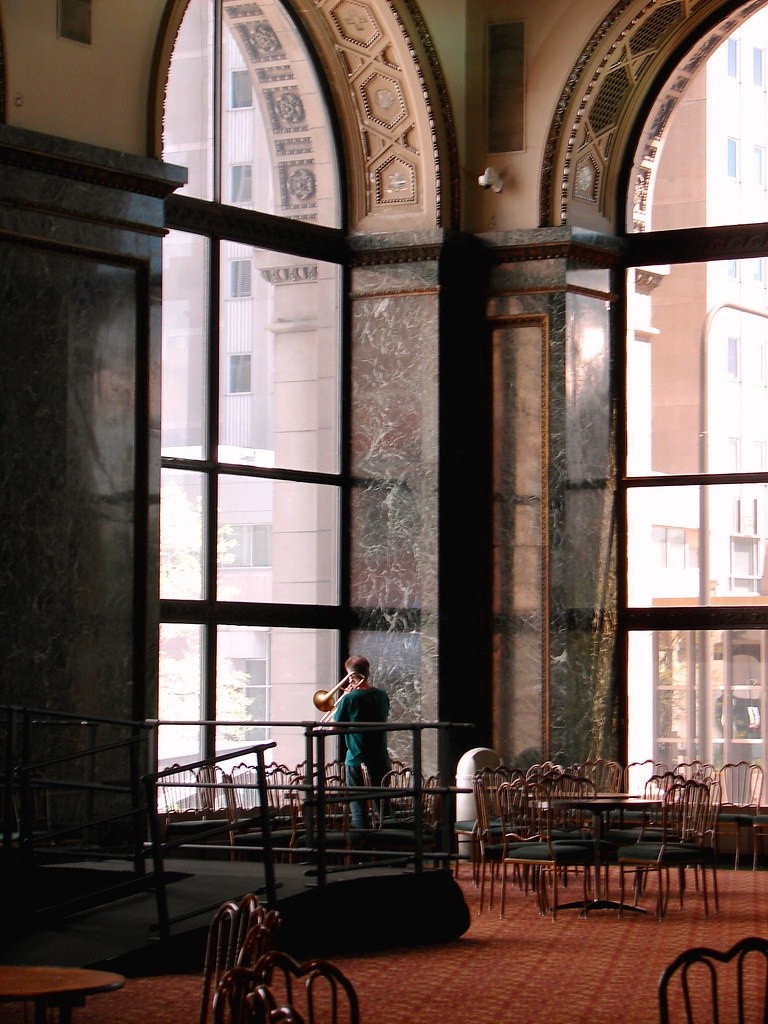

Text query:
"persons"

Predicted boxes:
[[331, 655, 395, 829]]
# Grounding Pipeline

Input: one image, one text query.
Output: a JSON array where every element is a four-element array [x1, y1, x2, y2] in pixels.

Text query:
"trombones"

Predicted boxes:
[[313, 671, 366, 730]]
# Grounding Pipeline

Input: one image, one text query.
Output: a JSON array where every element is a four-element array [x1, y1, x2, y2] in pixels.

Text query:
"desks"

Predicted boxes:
[[537, 799, 673, 918], [0, 966, 125, 1024]]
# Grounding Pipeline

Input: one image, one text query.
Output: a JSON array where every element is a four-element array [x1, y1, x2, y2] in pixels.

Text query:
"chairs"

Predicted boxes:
[[162, 758, 768, 1024]]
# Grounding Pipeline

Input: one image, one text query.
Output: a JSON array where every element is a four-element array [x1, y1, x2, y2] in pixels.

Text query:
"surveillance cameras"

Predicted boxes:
[[478, 175, 494, 189]]
